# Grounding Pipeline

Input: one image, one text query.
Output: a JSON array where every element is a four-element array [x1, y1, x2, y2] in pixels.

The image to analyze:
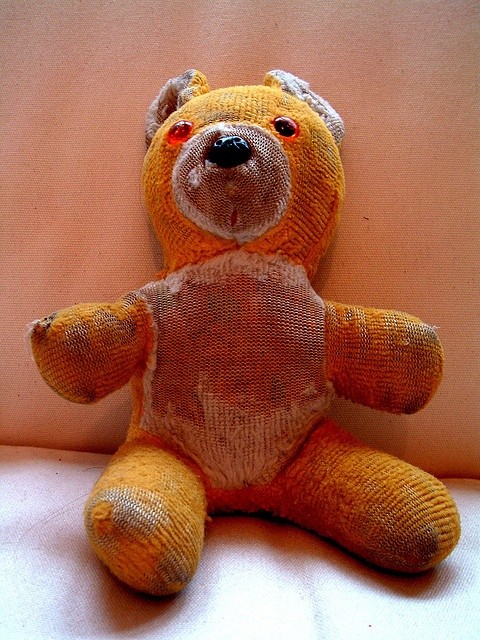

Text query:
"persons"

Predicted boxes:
[[29, 69, 462, 599]]
